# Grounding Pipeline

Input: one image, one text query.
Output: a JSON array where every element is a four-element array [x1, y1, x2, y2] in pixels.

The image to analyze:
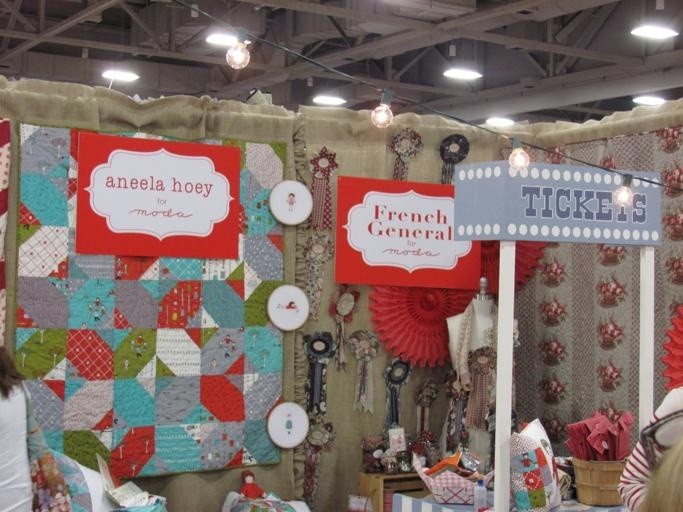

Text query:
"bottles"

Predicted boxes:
[[474, 479, 488, 511]]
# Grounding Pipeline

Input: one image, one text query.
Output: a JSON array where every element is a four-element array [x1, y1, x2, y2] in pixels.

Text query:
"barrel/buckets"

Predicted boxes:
[[570, 455, 630, 507]]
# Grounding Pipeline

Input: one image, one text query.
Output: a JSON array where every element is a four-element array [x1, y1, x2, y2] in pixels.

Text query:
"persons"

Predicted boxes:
[[642, 438, 683, 512], [617, 386, 683, 512], [0, 345, 35, 511]]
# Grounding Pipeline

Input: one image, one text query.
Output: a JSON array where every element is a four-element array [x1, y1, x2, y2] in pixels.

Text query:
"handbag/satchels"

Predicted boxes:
[[18, 379, 72, 512]]
